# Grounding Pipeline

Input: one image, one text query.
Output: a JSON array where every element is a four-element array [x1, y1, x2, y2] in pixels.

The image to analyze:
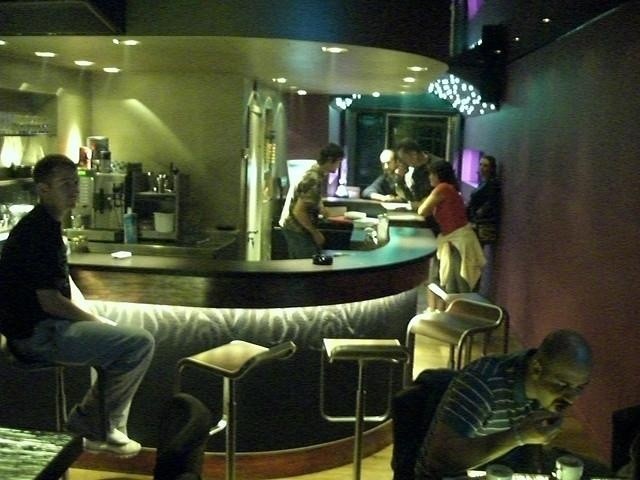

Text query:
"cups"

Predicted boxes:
[[550, 454, 584, 480], [485, 463, 513, 480]]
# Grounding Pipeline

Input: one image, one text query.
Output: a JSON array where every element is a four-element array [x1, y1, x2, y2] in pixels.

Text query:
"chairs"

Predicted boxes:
[[390, 365, 458, 479], [152, 392, 211, 480], [610, 406, 639, 477]]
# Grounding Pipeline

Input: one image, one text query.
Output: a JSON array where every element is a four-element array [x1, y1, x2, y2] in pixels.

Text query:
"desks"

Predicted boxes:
[[462, 442, 611, 480], [0, 422, 84, 480]]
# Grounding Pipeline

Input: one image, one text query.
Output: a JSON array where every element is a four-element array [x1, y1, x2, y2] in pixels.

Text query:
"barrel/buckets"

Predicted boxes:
[[153, 212, 175, 233]]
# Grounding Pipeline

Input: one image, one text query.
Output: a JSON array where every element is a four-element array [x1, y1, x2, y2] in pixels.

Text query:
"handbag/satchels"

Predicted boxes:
[[476, 219, 497, 244]]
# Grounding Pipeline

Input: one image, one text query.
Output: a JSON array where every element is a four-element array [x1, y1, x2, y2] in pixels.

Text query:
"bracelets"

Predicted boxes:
[[513, 424, 525, 448]]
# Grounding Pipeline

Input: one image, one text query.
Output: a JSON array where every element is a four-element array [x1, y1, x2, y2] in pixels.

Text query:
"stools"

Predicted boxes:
[[427, 282, 510, 368], [403, 298, 504, 389], [166, 334, 297, 480], [1, 338, 66, 432], [317, 335, 413, 479]]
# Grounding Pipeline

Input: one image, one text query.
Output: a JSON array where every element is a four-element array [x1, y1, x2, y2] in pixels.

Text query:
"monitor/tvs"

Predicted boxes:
[[459, 146, 484, 188], [286, 157, 319, 190]]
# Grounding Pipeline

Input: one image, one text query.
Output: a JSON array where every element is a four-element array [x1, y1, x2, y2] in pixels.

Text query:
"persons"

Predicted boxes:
[[413, 329, 593, 480], [0, 152, 155, 460], [279, 142, 344, 259], [361, 140, 500, 313]]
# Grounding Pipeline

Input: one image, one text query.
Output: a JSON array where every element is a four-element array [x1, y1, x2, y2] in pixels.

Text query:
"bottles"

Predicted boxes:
[[79, 204, 93, 230], [122, 206, 139, 243]]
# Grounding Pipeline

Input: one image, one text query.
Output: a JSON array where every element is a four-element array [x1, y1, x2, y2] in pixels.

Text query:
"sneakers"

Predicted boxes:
[[83, 437, 142, 459], [67, 405, 130, 446]]
